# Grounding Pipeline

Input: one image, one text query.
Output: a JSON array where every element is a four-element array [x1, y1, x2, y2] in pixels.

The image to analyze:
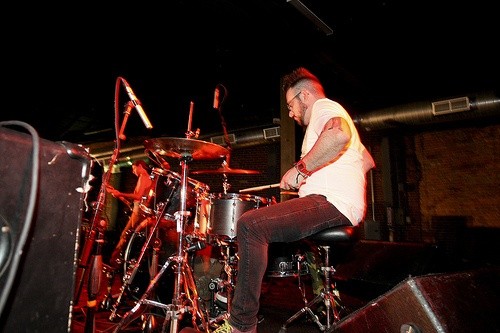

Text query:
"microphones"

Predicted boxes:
[[212, 86, 220, 110], [122, 79, 153, 129]]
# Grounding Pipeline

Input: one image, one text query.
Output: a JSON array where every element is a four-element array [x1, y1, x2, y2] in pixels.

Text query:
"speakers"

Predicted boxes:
[[0, 126, 92, 333], [325, 267, 500, 333]]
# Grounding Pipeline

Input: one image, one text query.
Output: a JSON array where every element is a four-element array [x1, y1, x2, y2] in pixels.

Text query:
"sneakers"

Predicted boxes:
[[211, 318, 256, 333]]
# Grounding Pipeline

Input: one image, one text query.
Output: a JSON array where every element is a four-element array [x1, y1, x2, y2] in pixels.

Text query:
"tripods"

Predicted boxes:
[[109, 158, 212, 332]]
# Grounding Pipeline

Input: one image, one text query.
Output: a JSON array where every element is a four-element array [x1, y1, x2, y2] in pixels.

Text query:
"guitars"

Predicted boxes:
[[106, 185, 134, 214]]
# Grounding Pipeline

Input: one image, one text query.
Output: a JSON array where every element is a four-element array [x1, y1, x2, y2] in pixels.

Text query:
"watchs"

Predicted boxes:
[[296, 160, 313, 177]]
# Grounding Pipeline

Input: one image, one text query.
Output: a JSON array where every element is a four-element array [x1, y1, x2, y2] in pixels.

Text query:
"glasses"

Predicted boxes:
[[286, 91, 309, 110]]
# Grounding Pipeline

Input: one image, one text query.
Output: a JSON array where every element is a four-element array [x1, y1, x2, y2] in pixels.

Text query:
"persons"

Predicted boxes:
[[211, 67, 375, 333]]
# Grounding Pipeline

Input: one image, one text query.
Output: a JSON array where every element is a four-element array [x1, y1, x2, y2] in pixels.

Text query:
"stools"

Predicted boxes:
[[279, 225, 357, 333]]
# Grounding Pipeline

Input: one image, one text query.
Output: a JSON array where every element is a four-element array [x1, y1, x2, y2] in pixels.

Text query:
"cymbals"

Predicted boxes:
[[144, 137, 229, 160], [189, 167, 262, 174]]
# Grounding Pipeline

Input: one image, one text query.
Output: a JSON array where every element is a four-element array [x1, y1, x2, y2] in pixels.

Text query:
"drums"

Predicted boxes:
[[265, 242, 308, 277], [138, 167, 210, 224], [207, 193, 269, 243], [117, 217, 237, 314], [193, 191, 210, 241]]
